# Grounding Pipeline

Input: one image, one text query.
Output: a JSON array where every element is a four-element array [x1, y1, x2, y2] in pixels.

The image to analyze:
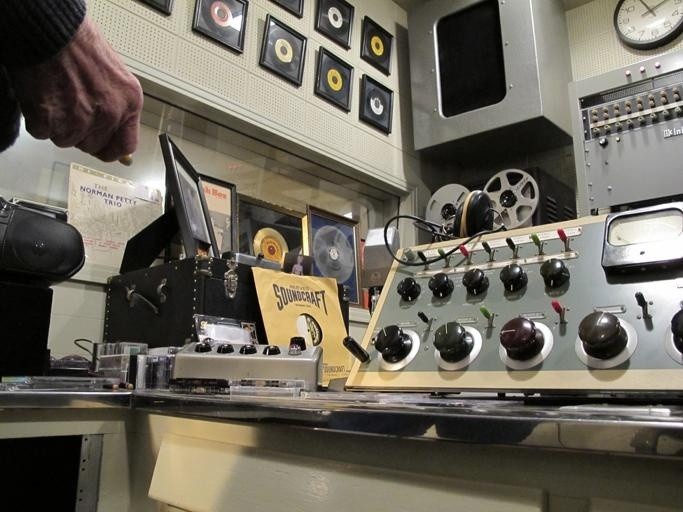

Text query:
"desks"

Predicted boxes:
[[1, 388, 683, 512]]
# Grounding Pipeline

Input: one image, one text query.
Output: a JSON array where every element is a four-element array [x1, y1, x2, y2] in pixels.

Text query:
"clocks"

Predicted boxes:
[[613, 0, 683, 49]]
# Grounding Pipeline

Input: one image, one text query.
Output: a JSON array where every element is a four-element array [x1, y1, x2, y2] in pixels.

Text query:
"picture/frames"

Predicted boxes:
[[315, 0, 354, 50], [274, 0, 304, 18], [361, 16, 394, 76], [144, 0, 173, 14], [164, 173, 363, 309], [258, 14, 307, 86], [359, 74, 394, 134], [192, 0, 249, 54], [314, 46, 353, 112]]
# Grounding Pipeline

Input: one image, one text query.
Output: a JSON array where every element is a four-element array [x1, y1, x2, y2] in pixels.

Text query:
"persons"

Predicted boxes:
[[0, 0, 144, 164]]
